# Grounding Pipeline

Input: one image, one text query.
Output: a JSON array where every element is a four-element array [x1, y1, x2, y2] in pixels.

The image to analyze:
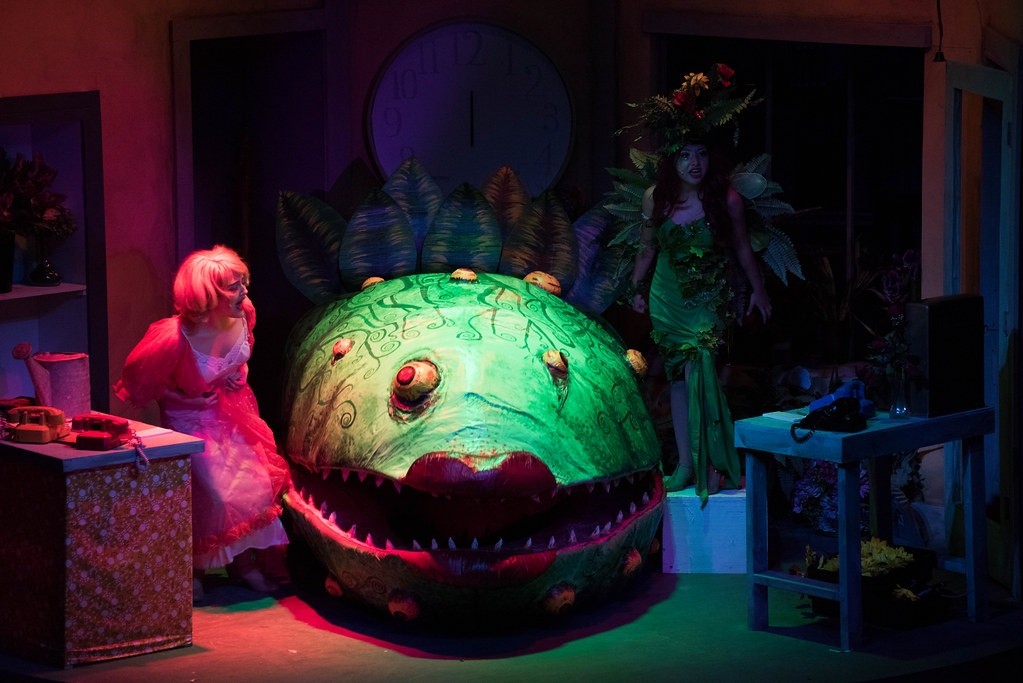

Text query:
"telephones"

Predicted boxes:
[[800, 397, 867, 433], [55, 413, 137, 451], [7, 406, 72, 444], [809, 380, 875, 421]]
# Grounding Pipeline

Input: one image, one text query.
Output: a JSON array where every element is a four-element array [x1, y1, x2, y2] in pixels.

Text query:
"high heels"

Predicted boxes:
[[663, 462, 695, 493], [721, 476, 738, 495]]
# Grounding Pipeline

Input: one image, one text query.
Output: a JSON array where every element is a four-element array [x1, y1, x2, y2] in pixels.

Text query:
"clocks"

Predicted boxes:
[[362, 14, 577, 203]]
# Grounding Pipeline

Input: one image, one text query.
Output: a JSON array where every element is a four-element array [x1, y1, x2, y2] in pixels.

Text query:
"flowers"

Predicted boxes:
[[859, 250, 920, 389], [613, 63, 765, 153], [786, 537, 920, 618], [0, 141, 70, 232]]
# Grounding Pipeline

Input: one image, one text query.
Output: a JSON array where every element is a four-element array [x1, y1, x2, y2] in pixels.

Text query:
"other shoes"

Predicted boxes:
[[225, 561, 292, 595]]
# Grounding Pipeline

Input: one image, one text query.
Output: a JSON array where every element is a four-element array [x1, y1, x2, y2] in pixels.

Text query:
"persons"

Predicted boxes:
[[112, 245, 292, 604], [629, 140, 776, 499]]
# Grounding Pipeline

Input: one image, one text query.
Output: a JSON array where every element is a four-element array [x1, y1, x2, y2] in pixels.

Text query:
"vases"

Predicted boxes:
[[0, 230, 15, 293], [808, 551, 915, 618], [886, 366, 910, 417]]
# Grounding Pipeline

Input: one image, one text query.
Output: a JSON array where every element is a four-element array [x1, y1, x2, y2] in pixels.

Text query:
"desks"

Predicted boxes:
[[734, 406, 996, 650], [0, 409, 205, 671]]
